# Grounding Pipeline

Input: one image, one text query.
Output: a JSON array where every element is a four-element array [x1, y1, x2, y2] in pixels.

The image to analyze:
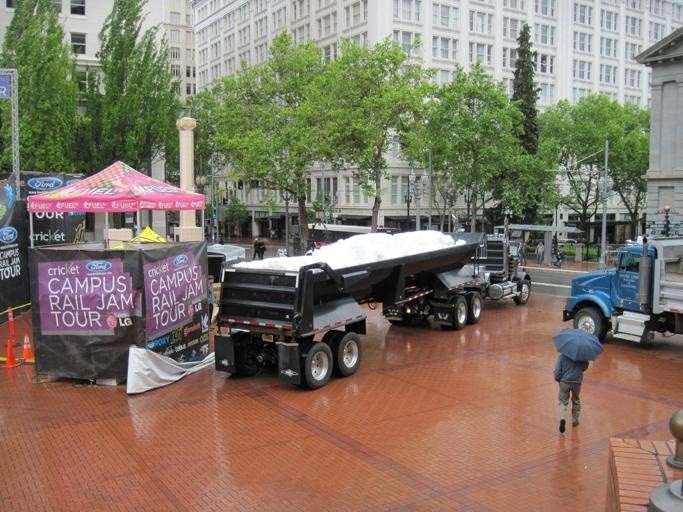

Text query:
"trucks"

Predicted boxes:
[[563, 235, 683, 343], [214, 230, 531, 390]]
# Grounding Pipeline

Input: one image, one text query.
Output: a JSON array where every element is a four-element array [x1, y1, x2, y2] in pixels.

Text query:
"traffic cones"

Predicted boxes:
[[0, 338, 21, 369], [22, 333, 36, 363]]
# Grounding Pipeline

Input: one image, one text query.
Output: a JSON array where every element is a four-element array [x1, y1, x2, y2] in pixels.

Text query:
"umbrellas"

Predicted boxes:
[[553, 328, 605, 363]]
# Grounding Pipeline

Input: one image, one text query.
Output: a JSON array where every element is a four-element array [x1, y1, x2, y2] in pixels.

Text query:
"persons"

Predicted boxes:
[[258, 240, 266, 259], [293, 234, 300, 256], [554, 353, 589, 433], [253, 238, 259, 258], [535, 241, 544, 265], [219, 238, 224, 245], [516, 243, 527, 266]]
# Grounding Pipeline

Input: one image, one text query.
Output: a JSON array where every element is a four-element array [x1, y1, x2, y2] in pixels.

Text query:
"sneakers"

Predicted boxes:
[[573, 422, 578, 426], [560, 419, 566, 432]]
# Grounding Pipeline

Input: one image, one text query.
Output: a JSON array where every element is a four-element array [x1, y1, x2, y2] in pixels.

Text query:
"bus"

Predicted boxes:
[[307, 223, 401, 243]]
